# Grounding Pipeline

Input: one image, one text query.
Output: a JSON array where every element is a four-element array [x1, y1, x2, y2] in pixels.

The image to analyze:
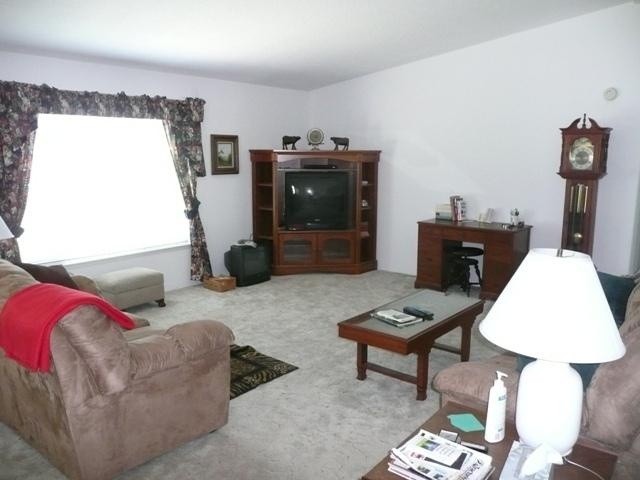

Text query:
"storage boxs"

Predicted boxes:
[[203, 274, 236, 292]]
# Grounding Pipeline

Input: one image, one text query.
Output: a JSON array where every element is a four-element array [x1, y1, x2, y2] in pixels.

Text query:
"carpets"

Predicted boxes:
[[230, 342, 298, 401]]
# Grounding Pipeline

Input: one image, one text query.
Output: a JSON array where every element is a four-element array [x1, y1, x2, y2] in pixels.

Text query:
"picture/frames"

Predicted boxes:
[[210, 134, 239, 174]]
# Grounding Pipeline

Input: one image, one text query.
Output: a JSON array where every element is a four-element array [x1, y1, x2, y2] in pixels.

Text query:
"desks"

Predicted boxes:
[[415, 222, 533, 301]]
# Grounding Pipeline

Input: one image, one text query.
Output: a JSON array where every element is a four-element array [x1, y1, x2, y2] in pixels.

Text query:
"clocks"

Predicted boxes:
[[307, 128, 324, 151], [556, 113, 612, 256]]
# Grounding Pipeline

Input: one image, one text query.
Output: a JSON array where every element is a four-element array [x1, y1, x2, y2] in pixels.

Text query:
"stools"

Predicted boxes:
[[94, 267, 166, 310], [440, 246, 484, 292]]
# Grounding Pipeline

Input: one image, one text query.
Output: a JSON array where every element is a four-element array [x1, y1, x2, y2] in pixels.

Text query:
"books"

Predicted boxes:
[[369, 307, 422, 328], [377, 309, 416, 323], [450, 194, 468, 223], [387, 428, 497, 480]]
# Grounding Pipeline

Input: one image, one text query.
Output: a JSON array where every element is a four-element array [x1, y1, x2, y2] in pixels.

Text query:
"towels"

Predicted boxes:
[[0, 283, 134, 373]]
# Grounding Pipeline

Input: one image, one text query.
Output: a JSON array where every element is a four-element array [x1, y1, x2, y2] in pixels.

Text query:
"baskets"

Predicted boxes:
[[203, 276, 236, 292]]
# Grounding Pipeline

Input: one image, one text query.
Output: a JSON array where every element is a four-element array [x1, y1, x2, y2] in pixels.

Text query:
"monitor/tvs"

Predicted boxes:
[[285, 172, 347, 230], [225, 243, 272, 286]]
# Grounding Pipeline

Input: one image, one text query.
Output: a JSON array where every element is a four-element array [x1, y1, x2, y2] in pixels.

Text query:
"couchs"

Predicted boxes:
[[0, 257, 234, 480], [431, 270, 640, 455]]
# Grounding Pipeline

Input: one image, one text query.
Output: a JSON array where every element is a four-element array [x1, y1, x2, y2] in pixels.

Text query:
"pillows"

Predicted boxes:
[[10, 261, 80, 290], [596, 271, 635, 329], [514, 355, 599, 392]]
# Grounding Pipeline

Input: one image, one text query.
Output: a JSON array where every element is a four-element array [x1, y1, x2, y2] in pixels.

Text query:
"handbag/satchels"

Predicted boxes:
[[231, 241, 271, 286]]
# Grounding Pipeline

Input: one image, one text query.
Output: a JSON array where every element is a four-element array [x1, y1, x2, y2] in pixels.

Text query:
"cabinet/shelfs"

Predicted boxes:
[[278, 230, 357, 275], [249, 149, 278, 276], [357, 150, 381, 275]]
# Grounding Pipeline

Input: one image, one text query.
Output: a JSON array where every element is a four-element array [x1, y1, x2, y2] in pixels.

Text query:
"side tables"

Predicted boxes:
[[360, 400, 618, 480]]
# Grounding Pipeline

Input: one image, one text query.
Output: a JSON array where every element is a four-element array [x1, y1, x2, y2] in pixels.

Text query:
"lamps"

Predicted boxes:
[[477, 247, 628, 461]]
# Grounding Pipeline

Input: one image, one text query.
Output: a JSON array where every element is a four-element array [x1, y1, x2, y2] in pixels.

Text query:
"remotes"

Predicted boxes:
[[415, 308, 434, 317], [403, 306, 426, 317]]
